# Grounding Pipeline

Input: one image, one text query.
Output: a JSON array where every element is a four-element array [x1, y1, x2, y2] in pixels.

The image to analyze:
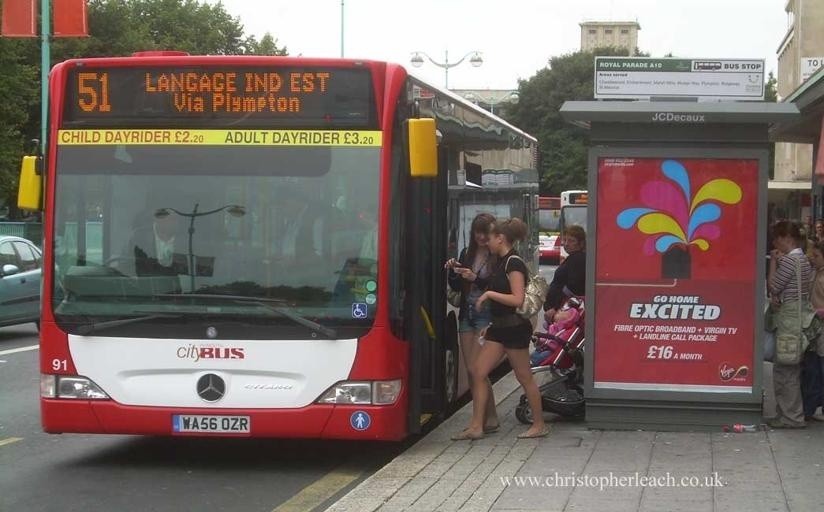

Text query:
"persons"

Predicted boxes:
[[543, 224, 585, 331], [122, 203, 189, 278], [266, 183, 330, 288], [764, 217, 824, 428], [442, 213, 501, 436], [449, 217, 549, 441]]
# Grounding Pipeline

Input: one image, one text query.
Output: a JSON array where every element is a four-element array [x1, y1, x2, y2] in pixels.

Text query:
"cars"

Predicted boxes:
[[0, 236, 42, 332]]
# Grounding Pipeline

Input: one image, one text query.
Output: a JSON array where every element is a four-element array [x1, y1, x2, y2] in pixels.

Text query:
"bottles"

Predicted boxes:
[[733, 422, 774, 434]]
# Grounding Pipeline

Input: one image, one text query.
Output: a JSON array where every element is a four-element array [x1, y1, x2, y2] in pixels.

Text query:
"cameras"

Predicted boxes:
[[452, 265, 468, 274]]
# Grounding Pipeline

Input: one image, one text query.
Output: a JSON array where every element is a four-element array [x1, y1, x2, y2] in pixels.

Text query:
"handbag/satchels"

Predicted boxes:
[[514, 274, 550, 321], [774, 332, 805, 366], [447, 287, 464, 308]]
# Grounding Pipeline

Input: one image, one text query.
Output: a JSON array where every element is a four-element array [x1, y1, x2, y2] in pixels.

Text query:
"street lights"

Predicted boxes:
[[409, 50, 520, 114]]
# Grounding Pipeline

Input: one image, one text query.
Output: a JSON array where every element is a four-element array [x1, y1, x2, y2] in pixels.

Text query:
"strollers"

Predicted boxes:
[[517, 294, 585, 424]]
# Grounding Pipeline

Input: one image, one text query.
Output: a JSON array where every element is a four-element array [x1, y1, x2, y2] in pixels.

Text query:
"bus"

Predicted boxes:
[[539, 189, 588, 266], [16, 50, 539, 443]]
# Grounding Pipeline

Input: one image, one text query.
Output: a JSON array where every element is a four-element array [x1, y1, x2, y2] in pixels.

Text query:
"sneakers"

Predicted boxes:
[[765, 415, 807, 429], [812, 407, 824, 421]]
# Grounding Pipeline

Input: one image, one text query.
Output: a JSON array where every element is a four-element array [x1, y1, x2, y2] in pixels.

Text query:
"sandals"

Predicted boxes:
[[463, 424, 501, 433], [517, 423, 552, 439], [450, 430, 486, 440]]
